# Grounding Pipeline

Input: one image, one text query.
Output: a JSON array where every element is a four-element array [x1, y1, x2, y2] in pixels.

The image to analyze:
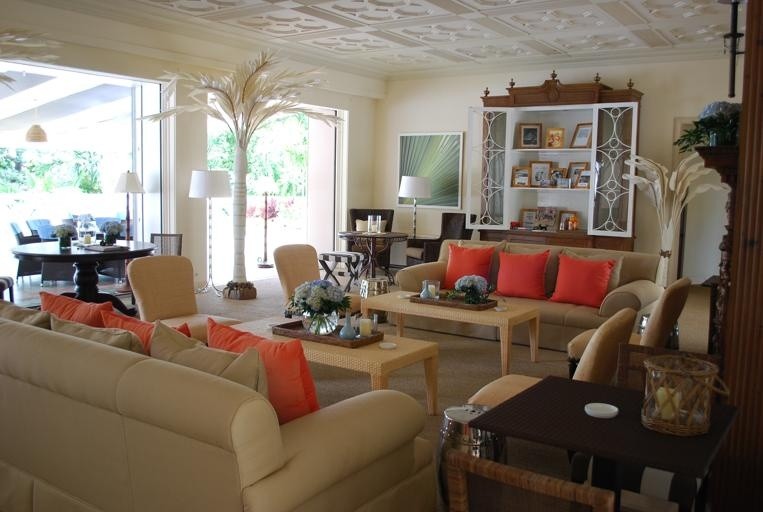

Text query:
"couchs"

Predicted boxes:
[[0, 297, 438, 511], [396, 240, 664, 353]]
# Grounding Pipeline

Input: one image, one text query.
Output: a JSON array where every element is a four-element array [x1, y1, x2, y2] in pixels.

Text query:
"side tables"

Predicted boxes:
[[441, 375, 739, 511]]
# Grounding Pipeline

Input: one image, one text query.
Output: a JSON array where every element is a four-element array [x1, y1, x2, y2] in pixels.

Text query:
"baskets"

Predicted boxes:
[[640, 355, 730, 437]]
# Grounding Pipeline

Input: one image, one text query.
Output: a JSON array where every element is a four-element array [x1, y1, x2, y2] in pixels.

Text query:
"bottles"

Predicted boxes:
[[558, 217, 567, 231], [568, 216, 576, 230], [419, 280, 432, 299], [339, 308, 356, 340]]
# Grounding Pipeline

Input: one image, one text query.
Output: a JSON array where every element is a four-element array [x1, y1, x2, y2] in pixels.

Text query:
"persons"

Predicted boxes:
[[534, 214, 557, 229], [549, 132, 555, 142]]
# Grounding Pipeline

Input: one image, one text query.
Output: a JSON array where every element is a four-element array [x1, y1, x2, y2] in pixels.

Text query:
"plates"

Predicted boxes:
[[584, 403, 620, 418], [379, 342, 396, 349]]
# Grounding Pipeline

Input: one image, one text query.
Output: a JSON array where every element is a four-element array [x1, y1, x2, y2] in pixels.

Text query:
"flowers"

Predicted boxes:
[[286, 281, 351, 319], [455, 275, 489, 293]]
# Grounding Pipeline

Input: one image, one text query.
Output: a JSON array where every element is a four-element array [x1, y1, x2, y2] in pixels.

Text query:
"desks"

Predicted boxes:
[[339, 231, 408, 286]]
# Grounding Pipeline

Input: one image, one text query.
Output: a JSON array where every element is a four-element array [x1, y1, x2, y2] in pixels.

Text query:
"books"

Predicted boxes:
[[85, 243, 128, 252]]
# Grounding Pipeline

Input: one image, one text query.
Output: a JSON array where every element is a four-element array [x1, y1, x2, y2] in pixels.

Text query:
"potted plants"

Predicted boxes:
[[671, 113, 740, 154]]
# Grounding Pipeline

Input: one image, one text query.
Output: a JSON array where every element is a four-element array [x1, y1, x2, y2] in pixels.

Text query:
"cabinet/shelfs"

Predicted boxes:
[[480, 70, 644, 239]]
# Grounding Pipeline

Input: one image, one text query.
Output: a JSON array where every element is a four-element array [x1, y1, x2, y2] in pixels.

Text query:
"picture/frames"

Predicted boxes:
[[397, 132, 465, 210], [511, 122, 592, 232]]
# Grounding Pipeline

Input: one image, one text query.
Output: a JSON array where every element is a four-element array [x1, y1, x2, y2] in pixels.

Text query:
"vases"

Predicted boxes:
[[465, 291, 483, 304], [301, 307, 338, 335]]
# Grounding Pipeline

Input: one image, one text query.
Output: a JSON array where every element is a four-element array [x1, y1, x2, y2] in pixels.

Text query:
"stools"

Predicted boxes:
[[317, 251, 363, 293]]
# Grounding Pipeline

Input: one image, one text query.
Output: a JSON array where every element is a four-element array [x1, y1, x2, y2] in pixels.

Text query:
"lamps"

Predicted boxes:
[[188, 169, 233, 297], [398, 175, 430, 269], [116, 172, 146, 293], [25, 99, 47, 142]]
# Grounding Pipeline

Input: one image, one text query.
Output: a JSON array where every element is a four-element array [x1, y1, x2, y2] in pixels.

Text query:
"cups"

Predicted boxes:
[[77, 220, 95, 244], [367, 215, 373, 233], [422, 281, 439, 299], [376, 216, 381, 233], [354, 314, 378, 339]]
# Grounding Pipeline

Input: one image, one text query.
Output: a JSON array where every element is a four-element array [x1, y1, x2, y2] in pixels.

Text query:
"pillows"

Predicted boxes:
[[443, 244, 625, 308], [101, 307, 192, 354], [355, 219, 388, 232], [50, 313, 145, 356], [39, 290, 113, 327], [206, 317, 318, 424], [1, 299, 51, 329], [150, 320, 268, 398]]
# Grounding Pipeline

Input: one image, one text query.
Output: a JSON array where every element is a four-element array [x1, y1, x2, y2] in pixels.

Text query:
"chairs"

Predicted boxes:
[[0, 215, 183, 316], [406, 213, 477, 265], [126, 256, 241, 344], [465, 308, 637, 408], [568, 279, 693, 379], [349, 208, 394, 275], [273, 244, 364, 315]]
